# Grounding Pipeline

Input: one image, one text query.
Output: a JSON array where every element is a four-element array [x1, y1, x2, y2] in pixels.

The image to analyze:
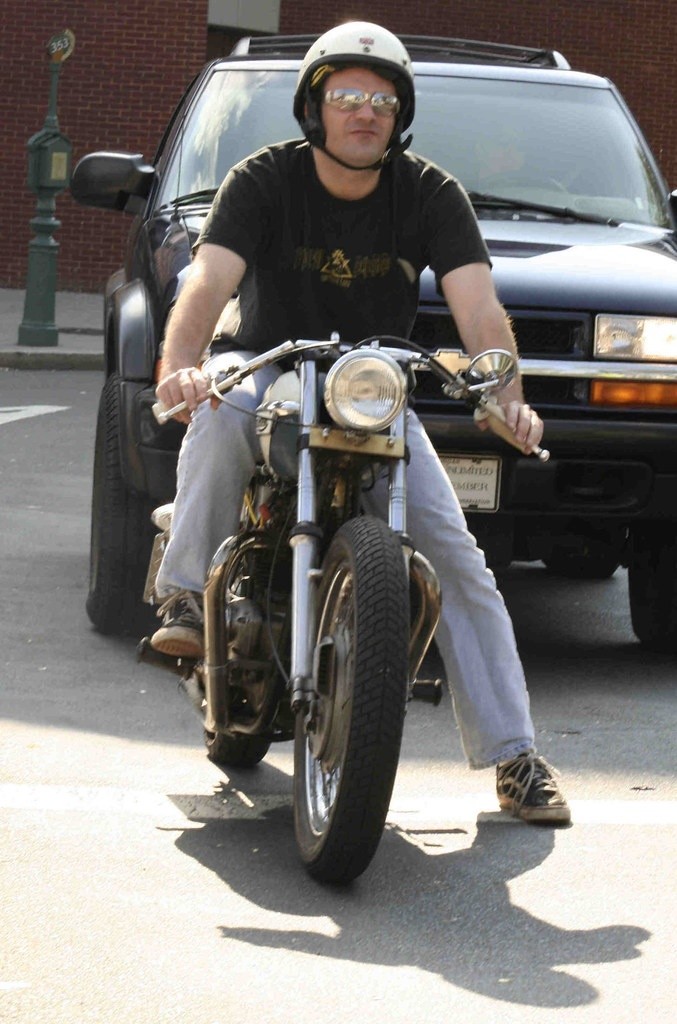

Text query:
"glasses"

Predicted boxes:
[[311, 87, 401, 119]]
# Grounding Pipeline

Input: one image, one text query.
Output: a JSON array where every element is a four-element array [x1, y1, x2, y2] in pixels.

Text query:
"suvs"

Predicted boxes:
[[66, 32, 677, 656]]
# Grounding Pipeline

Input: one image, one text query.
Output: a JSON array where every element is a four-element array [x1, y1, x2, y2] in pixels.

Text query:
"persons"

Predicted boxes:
[[149, 16, 571, 823]]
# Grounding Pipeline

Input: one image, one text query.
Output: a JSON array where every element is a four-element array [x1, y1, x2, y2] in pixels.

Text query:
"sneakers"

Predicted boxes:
[[152, 589, 203, 658], [496, 748, 569, 821]]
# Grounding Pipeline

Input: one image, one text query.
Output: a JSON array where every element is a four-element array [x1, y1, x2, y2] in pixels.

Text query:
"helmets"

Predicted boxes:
[[292, 21, 417, 139]]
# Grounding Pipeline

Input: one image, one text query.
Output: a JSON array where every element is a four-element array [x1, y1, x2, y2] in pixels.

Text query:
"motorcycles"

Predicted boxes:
[[135, 330, 551, 890]]
